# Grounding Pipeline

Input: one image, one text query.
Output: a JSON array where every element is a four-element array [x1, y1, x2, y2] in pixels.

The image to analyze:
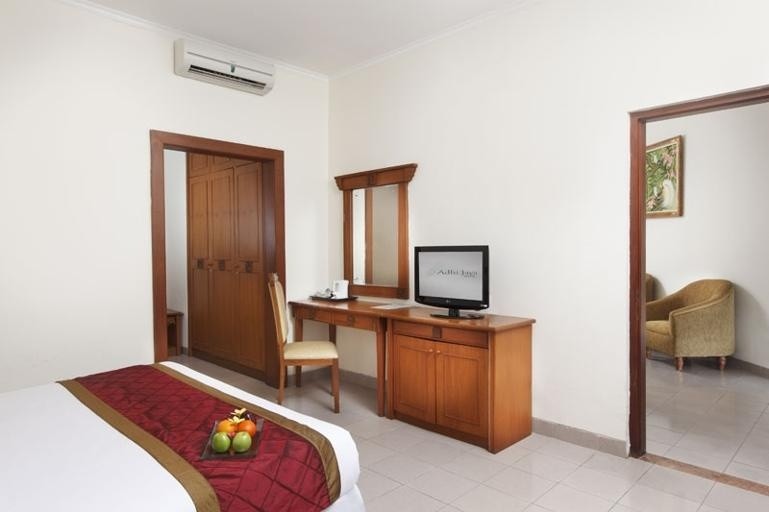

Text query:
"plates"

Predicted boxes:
[[201, 419, 263, 460]]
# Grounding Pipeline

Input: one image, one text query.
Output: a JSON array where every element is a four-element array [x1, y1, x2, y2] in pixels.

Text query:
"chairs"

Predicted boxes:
[[266, 272, 340, 414]]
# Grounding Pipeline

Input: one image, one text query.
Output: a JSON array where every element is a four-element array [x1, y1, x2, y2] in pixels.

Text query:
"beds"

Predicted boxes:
[[0, 360, 365, 512]]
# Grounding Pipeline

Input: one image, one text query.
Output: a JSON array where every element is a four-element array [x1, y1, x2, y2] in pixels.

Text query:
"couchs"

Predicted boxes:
[[646, 279, 735, 371], [646, 274, 654, 302]]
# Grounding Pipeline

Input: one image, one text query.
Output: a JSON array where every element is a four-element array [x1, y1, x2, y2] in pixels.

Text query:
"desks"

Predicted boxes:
[[288, 300, 413, 417]]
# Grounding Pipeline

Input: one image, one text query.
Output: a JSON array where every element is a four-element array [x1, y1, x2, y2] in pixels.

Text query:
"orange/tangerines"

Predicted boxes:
[[232, 432, 252, 452], [211, 433, 230, 452]]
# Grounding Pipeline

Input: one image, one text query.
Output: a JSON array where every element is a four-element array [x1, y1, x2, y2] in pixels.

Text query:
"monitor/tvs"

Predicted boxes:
[[414, 245, 489, 321]]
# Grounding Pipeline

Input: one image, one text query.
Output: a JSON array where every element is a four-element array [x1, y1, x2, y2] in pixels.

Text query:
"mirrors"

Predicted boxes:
[[333, 162, 418, 300]]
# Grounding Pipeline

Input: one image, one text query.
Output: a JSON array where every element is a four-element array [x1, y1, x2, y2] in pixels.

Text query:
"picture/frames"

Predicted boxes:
[[645, 134, 684, 218]]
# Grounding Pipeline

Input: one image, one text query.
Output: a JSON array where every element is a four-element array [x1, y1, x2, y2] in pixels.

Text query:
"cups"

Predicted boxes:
[[332, 279, 349, 299]]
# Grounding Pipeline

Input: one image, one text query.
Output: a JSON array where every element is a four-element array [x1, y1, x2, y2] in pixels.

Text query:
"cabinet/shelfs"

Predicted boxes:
[[386, 317, 532, 454]]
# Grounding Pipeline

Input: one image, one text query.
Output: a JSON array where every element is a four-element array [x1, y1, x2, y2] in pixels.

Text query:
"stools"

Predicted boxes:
[[167, 309, 183, 355]]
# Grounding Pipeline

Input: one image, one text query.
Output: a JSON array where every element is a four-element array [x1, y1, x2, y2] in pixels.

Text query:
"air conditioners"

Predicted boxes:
[[174, 39, 275, 96]]
